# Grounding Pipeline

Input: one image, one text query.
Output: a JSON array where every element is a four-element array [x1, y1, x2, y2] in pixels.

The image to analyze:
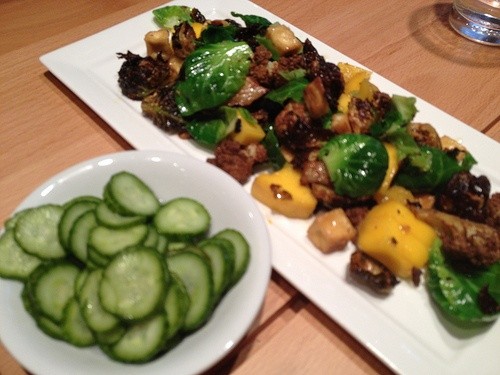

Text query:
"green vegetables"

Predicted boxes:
[[152, 4, 500, 325]]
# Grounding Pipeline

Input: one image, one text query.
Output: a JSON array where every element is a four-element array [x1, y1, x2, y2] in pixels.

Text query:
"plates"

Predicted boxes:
[[0, 148, 275, 375], [37, 0, 500, 375]]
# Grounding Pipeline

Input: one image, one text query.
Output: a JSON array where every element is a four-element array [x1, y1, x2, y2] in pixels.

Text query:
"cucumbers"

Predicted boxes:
[[0, 171, 250, 363]]
